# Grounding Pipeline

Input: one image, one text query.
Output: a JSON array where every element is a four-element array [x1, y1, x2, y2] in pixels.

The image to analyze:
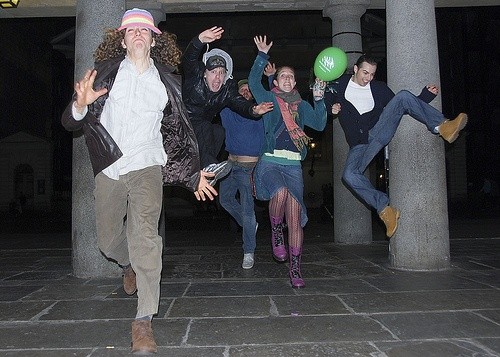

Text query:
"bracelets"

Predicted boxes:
[[313, 96, 323, 100]]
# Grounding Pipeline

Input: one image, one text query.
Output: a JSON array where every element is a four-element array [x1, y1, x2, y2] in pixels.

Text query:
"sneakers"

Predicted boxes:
[[242, 252, 255, 269]]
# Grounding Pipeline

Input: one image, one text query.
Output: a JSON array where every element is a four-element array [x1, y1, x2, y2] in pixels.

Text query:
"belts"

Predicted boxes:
[[229, 155, 259, 163]]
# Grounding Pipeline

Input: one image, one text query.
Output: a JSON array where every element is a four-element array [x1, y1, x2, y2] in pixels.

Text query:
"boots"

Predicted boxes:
[[269, 215, 288, 261], [289, 246, 306, 290]]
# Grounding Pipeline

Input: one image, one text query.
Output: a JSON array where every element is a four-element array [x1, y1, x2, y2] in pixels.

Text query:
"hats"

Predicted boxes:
[[206, 55, 228, 71], [115, 8, 163, 35], [238, 80, 250, 90]]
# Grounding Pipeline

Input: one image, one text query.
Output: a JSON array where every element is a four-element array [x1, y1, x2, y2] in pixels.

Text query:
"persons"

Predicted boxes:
[[324, 54, 468, 238], [218, 62, 278, 269], [182, 26, 274, 187], [248, 35, 327, 290], [61, 8, 218, 357]]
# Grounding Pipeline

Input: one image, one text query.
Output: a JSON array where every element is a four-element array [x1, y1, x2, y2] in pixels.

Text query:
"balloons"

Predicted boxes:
[[313, 47, 348, 82]]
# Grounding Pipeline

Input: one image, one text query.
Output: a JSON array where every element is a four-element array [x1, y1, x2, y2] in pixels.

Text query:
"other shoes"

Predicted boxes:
[[131, 320, 157, 355], [379, 207, 400, 237], [438, 112, 467, 144], [123, 264, 137, 295]]
